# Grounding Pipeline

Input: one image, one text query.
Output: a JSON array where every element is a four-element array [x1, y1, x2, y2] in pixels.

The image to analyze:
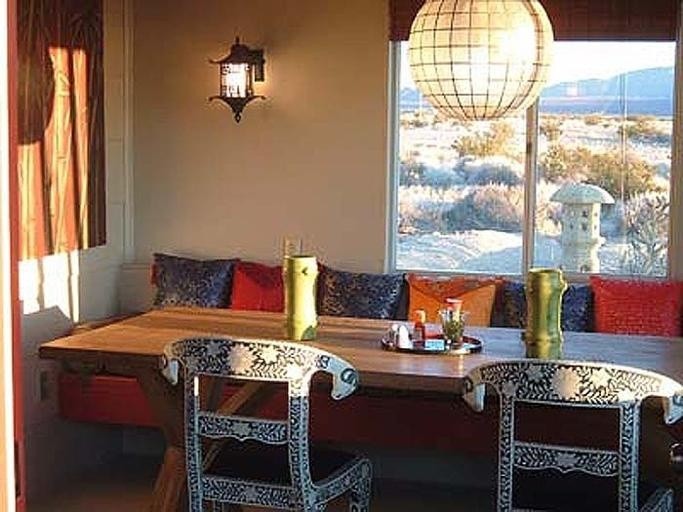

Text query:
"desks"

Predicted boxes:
[[40, 307, 682, 512]]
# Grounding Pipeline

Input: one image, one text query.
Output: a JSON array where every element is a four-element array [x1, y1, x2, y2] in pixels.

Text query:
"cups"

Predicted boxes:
[[438, 310, 470, 347]]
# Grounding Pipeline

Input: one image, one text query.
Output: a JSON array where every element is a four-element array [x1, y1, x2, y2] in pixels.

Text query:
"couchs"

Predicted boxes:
[[54, 311, 681, 467]]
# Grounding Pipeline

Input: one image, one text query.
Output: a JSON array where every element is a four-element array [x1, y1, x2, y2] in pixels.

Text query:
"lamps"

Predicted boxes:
[[408, 0, 554, 120], [206, 36, 267, 123]]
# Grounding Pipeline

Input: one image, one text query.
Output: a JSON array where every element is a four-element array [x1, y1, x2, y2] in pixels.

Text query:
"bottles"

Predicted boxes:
[[386, 309, 426, 350]]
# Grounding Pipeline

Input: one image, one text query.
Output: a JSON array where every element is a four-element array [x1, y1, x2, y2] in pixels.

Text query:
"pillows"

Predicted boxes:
[[229, 257, 284, 313], [405, 273, 497, 333], [314, 263, 405, 321], [589, 273, 683, 337], [492, 278, 590, 331], [151, 251, 233, 308]]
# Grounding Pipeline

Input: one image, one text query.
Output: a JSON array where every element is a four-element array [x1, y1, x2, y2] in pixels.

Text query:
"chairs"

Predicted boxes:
[[156, 334, 373, 512], [458, 359, 683, 511]]
